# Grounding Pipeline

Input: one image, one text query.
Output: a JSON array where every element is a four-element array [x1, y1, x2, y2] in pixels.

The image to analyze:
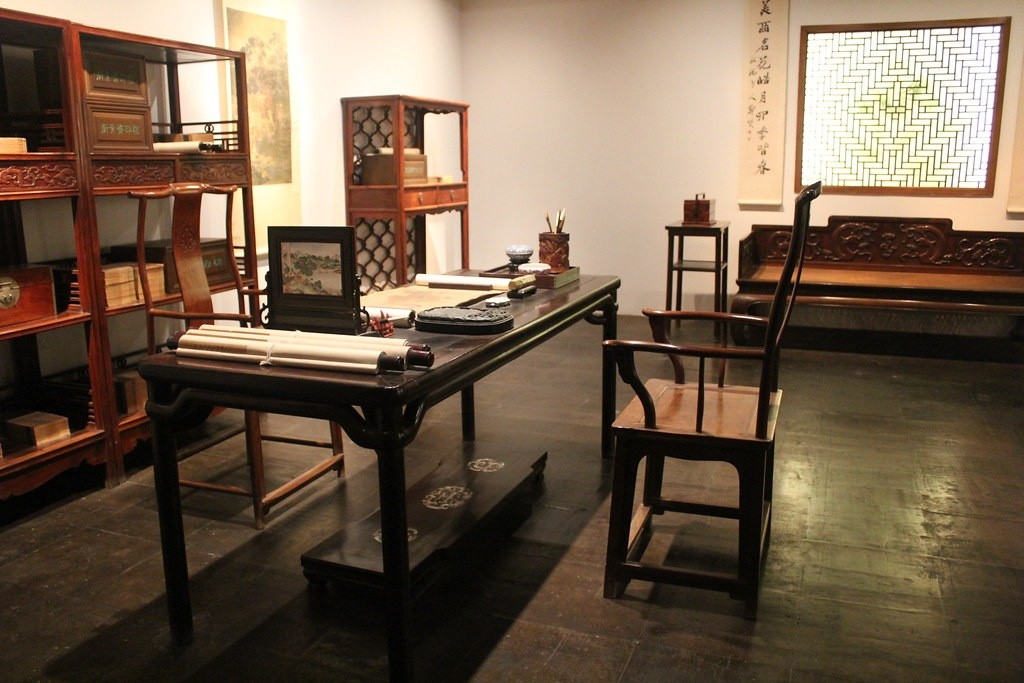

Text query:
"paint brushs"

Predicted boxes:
[[362, 315, 410, 327], [543, 207, 566, 233]]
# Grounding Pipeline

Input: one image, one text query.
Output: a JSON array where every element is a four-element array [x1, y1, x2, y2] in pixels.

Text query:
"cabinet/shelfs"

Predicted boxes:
[[0, 8, 260, 499], [340, 95, 469, 296]]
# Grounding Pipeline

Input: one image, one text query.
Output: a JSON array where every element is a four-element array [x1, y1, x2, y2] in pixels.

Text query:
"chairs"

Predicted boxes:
[[602, 182, 821, 620], [127, 181, 346, 531]]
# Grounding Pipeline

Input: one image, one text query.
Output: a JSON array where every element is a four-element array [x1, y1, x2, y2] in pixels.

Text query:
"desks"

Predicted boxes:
[[665, 222, 728, 339], [137, 268, 621, 683]]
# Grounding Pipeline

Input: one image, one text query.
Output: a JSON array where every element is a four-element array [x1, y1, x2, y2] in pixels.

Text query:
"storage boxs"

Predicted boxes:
[[33, 50, 154, 153], [0, 263, 57, 327], [113, 368, 148, 413], [111, 236, 234, 294], [361, 154, 428, 186]]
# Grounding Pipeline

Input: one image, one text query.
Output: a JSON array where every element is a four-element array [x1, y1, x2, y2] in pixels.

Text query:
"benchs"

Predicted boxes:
[[730, 215, 1023, 348]]
